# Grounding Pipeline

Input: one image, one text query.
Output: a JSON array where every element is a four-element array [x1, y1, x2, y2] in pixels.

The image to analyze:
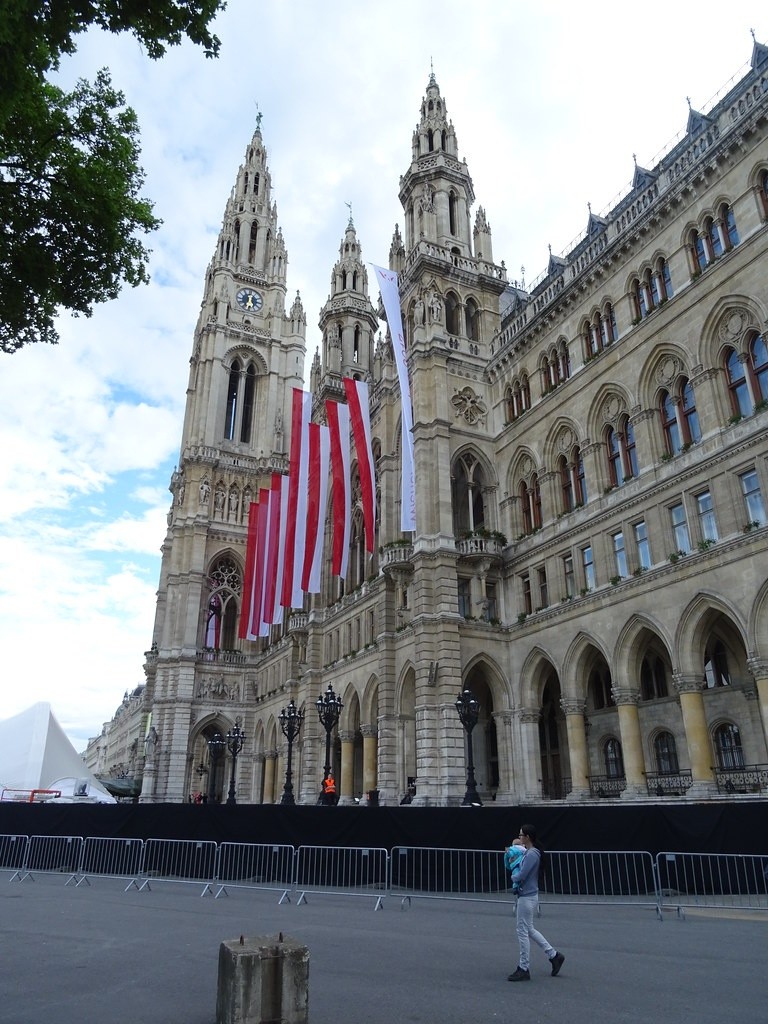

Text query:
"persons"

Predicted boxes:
[[143, 726, 158, 768], [504, 825, 565, 982], [188, 791, 208, 805], [322, 772, 336, 807], [199, 673, 239, 701]]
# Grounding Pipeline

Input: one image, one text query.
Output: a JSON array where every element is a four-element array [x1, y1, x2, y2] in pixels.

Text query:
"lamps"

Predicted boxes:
[[193, 731, 209, 781]]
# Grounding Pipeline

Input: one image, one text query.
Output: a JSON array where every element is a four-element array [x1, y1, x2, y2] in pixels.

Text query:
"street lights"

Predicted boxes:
[[278, 697, 306, 806], [455, 688, 485, 808], [225, 721, 248, 805], [315, 681, 344, 805], [205, 730, 227, 807]]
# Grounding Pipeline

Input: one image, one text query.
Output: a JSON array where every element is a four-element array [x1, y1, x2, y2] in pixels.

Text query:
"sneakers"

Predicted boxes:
[[508, 965, 530, 981], [549, 952, 565, 976]]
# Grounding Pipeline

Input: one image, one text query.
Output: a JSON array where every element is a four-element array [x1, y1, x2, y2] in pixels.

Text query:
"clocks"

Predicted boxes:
[[236, 288, 263, 312]]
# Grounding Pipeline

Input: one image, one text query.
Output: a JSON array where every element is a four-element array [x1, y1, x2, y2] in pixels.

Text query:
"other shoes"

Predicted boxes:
[[513, 889, 518, 895]]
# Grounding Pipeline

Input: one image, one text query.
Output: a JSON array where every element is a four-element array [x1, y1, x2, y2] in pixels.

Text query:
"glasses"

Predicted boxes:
[[518, 834, 526, 837]]
